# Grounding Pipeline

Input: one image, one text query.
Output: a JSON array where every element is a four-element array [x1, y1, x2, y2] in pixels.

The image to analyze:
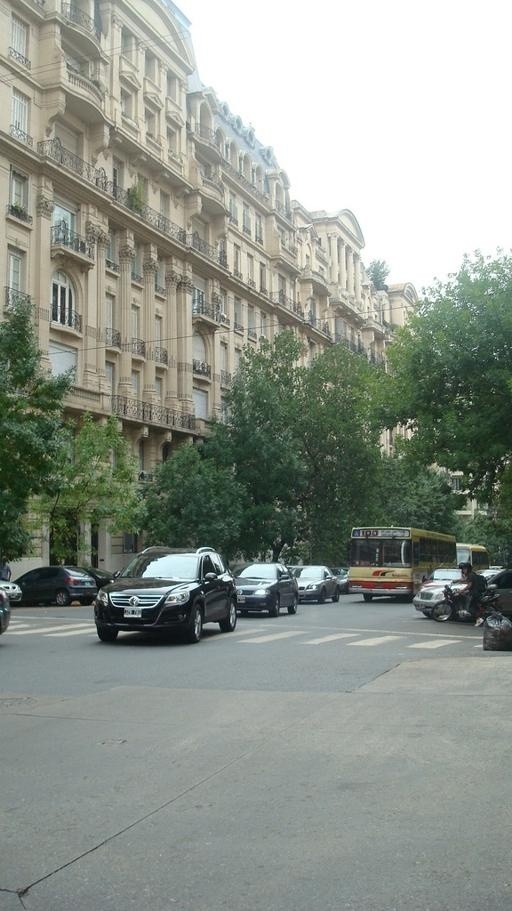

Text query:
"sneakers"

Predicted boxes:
[[474, 618, 484, 627]]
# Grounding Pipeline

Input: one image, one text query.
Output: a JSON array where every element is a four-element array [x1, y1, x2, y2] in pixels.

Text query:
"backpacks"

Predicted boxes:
[[470, 574, 487, 593]]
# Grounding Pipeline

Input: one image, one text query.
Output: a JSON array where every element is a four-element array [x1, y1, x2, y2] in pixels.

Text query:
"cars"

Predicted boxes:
[[412, 566, 512, 622], [94, 545, 349, 642], [0, 565, 120, 635]]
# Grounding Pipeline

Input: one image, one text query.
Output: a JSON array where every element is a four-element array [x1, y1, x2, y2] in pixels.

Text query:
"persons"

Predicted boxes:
[[0, 557, 12, 580], [452, 562, 484, 627]]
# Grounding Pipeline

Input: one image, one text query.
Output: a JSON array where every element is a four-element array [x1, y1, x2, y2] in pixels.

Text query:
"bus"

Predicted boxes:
[[348, 527, 489, 602]]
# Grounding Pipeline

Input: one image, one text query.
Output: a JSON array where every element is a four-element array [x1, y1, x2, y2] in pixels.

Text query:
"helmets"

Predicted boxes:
[[459, 562, 472, 575]]
